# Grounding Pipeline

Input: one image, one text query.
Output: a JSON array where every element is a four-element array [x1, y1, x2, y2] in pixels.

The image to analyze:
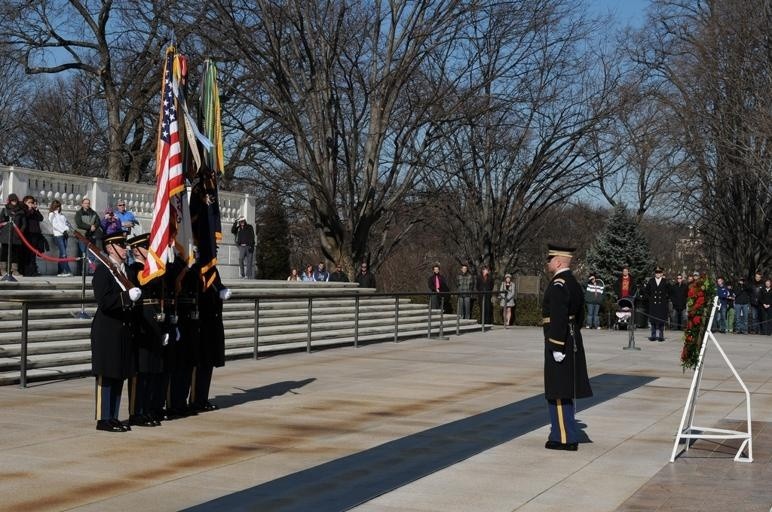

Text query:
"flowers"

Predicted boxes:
[[678, 279, 716, 372]]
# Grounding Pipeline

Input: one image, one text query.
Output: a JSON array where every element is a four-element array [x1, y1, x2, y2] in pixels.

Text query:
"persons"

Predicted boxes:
[[329, 264, 350, 282], [539, 241, 597, 452], [498, 273, 515, 329], [301, 263, 315, 281], [454, 263, 476, 319], [228, 214, 258, 280], [353, 262, 376, 288], [315, 261, 330, 282], [426, 264, 448, 313], [582, 266, 772, 341], [286, 267, 302, 281], [475, 266, 494, 324], [90, 232, 234, 433], [1, 194, 139, 277]]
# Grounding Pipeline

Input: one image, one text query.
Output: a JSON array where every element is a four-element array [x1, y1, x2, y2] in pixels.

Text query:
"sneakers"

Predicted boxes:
[[57, 272, 74, 277], [648, 336, 665, 342], [585, 325, 602, 330], [158, 403, 218, 421], [239, 276, 253, 280], [711, 329, 772, 337], [23, 271, 44, 276]]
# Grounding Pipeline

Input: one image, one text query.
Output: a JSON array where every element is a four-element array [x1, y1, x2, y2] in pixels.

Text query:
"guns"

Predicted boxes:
[[65, 221, 135, 292]]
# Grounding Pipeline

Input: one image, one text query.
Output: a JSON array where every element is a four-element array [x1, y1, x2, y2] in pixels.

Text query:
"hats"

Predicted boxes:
[[545, 242, 578, 259], [654, 266, 664, 273], [124, 232, 151, 250], [101, 230, 129, 246]]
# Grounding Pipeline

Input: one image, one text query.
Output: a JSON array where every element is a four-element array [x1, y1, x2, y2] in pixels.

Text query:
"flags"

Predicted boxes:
[[138, 38, 228, 295]]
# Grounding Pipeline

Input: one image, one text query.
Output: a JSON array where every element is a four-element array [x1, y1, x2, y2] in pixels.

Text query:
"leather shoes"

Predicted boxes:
[[128, 415, 153, 427], [96, 420, 123, 433], [110, 420, 130, 432], [144, 413, 161, 426], [544, 440, 578, 452]]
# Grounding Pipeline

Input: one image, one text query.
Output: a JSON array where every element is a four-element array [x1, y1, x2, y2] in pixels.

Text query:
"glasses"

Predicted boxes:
[[116, 203, 125, 206]]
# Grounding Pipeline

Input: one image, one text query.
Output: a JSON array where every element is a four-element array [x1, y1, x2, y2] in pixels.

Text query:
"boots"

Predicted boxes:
[[10, 262, 24, 278], [0, 261, 7, 275]]
